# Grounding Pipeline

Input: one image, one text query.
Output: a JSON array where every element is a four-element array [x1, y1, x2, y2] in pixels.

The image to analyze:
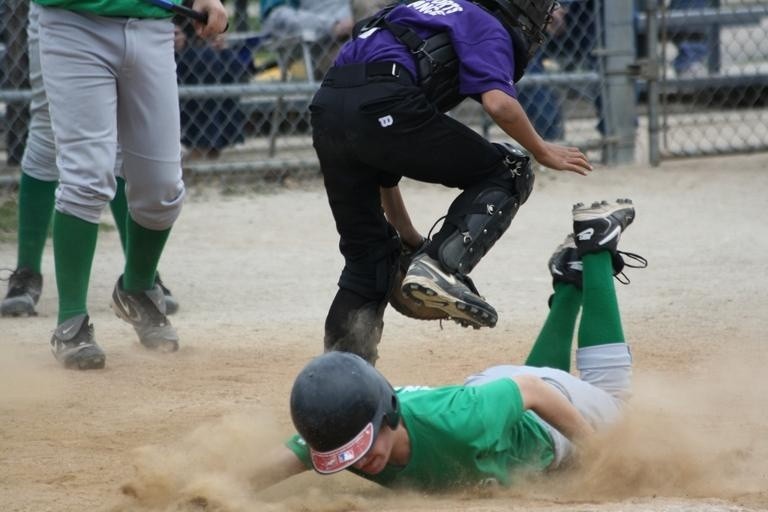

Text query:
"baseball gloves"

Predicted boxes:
[[389, 238, 449, 319]]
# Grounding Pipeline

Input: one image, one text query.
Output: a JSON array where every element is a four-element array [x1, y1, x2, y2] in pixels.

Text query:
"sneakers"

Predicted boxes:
[[108, 272, 181, 352], [0, 265, 42, 316], [399, 252, 498, 330], [549, 199, 648, 291], [50, 313, 105, 369], [677, 62, 708, 94]]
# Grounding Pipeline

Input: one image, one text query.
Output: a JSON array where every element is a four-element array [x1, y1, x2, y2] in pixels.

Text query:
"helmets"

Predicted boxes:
[[472, 1, 561, 84], [289, 351, 400, 475]]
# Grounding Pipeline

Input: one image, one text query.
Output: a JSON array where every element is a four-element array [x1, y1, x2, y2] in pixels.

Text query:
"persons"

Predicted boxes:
[[307, 0, 597, 372], [0, 1, 718, 163], [242, 198, 649, 497], [29, 0, 228, 367], [0, 1, 179, 320]]
